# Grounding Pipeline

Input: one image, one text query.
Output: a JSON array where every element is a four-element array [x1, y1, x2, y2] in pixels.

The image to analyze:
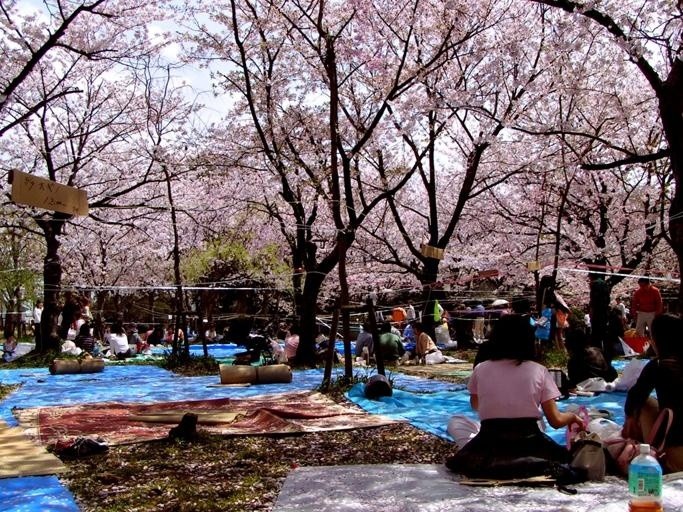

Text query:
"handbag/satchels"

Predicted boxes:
[[568, 402, 674, 483]]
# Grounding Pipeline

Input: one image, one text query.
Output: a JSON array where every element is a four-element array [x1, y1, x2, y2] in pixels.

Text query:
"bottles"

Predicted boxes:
[[626, 441, 668, 512]]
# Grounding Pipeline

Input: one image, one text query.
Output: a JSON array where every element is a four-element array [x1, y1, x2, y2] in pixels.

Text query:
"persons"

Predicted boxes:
[[445, 314, 586, 480], [2, 299, 301, 363], [622, 312, 683, 474]]
